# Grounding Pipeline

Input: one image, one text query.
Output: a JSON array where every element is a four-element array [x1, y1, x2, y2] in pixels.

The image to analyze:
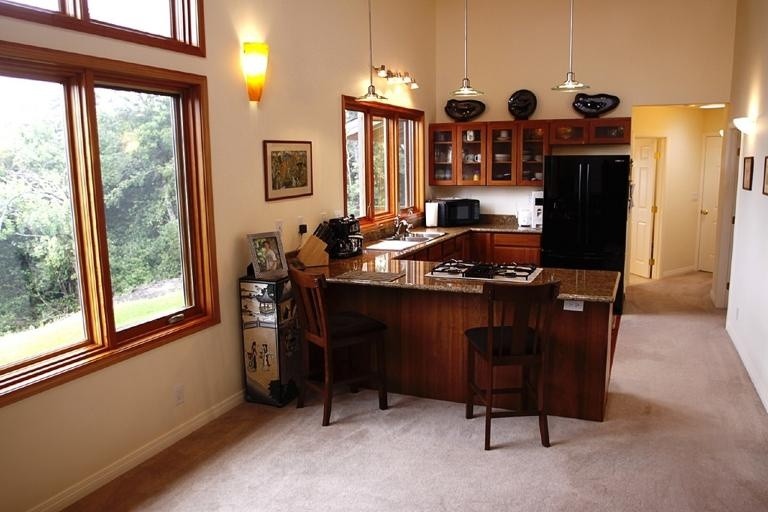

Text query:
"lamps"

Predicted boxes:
[[355, 0, 388, 102], [369, 60, 420, 90], [450, 1, 484, 97], [238, 44, 270, 105], [551, 0, 590, 91]]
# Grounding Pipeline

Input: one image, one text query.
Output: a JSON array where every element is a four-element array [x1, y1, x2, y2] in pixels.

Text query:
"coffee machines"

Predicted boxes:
[[329, 214, 364, 256]]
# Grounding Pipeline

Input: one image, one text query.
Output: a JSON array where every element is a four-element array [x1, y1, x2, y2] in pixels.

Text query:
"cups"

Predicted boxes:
[[501, 130, 508, 137], [464, 153, 481, 163]]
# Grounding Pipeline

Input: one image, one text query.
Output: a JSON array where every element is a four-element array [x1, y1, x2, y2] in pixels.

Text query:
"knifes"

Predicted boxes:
[[312, 221, 336, 244]]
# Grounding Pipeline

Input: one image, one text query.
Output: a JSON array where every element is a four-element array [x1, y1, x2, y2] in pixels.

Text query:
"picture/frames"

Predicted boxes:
[[762, 155, 768, 196], [742, 157, 754, 192], [263, 140, 313, 203], [246, 230, 290, 281]]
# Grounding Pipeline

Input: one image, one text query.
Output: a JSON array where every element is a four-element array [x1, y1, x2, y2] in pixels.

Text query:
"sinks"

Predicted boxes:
[[409, 233, 441, 238], [380, 237, 431, 242]]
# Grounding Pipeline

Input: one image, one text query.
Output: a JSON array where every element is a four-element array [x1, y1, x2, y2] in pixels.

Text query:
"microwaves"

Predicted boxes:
[[433, 198, 481, 227]]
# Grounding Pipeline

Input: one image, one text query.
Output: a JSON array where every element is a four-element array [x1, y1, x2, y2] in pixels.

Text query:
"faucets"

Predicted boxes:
[[395, 219, 408, 236], [405, 223, 415, 233]]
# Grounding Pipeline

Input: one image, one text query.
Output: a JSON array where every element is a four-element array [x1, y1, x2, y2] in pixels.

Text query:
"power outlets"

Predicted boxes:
[[297, 216, 306, 232]]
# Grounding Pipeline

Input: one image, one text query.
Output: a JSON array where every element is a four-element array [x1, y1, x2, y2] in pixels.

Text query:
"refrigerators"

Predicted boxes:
[[540, 154, 633, 317]]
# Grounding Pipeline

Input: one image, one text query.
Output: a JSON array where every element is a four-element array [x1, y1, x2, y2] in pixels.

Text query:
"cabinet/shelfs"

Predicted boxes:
[[429, 122, 487, 187], [492, 233, 541, 266], [238, 272, 308, 410], [468, 230, 493, 262], [392, 231, 469, 263], [550, 117, 632, 145], [488, 120, 549, 187]]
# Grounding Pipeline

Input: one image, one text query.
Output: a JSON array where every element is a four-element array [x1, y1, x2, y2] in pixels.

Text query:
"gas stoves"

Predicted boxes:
[[424, 257, 543, 284]]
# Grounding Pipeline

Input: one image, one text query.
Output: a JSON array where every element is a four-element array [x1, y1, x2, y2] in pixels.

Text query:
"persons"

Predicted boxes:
[[263, 241, 277, 271]]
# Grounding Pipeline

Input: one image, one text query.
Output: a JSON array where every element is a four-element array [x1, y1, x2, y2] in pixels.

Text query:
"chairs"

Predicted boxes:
[[283, 264, 389, 426], [463, 277, 562, 450]]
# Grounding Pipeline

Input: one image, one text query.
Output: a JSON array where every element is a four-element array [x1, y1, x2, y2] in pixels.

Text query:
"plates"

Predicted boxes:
[[507, 89, 538, 121], [494, 154, 511, 161], [496, 137, 509, 142]]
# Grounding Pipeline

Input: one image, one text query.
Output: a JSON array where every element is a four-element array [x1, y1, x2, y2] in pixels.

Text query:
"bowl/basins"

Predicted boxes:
[[535, 172, 543, 180], [523, 154, 530, 161], [535, 154, 542, 161]]
